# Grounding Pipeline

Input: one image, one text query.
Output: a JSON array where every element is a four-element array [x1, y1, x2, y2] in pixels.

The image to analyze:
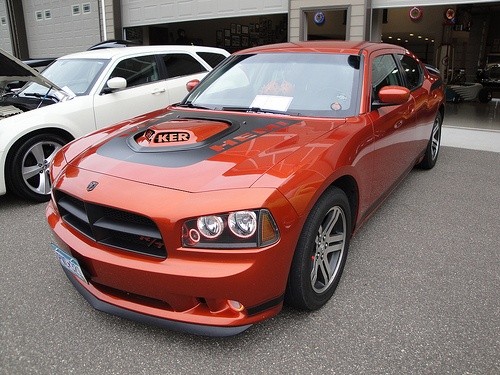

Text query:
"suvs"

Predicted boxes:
[[0, 44, 233, 203]]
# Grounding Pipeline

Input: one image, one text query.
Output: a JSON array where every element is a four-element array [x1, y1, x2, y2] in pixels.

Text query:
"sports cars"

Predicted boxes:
[[47, 40, 448, 337]]
